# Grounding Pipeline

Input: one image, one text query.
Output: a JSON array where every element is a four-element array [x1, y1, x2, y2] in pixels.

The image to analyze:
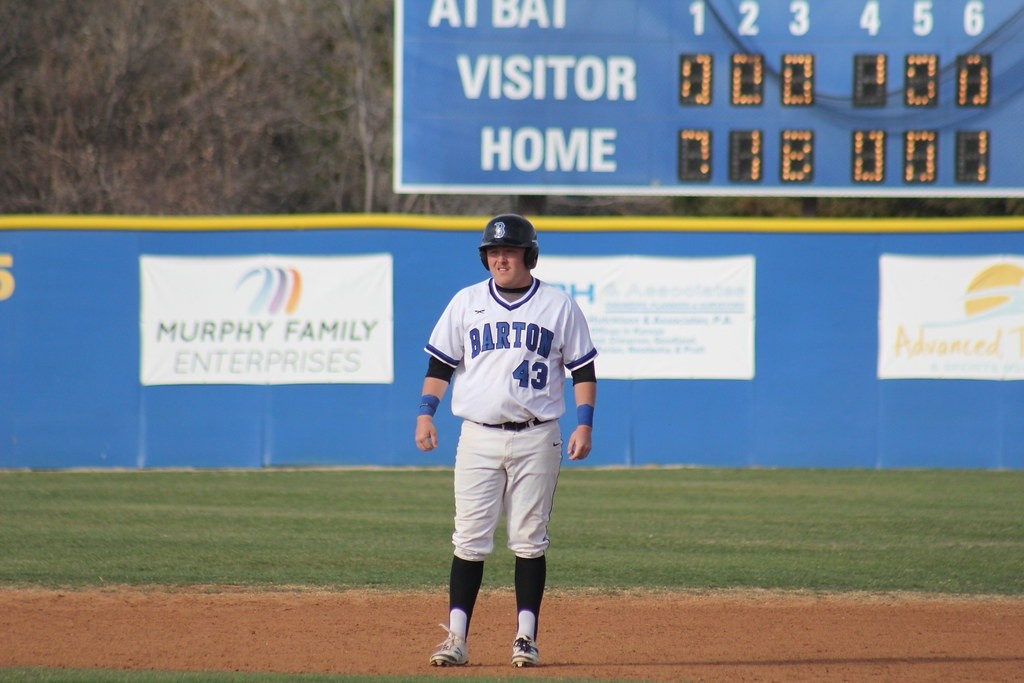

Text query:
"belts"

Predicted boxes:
[[475, 417, 552, 432]]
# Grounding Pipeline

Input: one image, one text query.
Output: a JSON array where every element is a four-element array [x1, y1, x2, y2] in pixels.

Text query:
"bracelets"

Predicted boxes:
[[577, 404, 594, 427], [417, 394, 440, 417], [420, 403, 436, 412]]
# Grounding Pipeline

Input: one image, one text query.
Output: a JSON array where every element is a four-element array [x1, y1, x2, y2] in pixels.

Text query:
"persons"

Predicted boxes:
[[416, 214, 597, 664]]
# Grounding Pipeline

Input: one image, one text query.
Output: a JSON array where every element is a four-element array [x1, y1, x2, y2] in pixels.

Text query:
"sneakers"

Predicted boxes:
[[511, 634, 539, 667], [430, 624, 469, 666]]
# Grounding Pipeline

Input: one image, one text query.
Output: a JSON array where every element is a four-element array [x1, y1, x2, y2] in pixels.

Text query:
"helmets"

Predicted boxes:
[[478, 214, 539, 271]]
